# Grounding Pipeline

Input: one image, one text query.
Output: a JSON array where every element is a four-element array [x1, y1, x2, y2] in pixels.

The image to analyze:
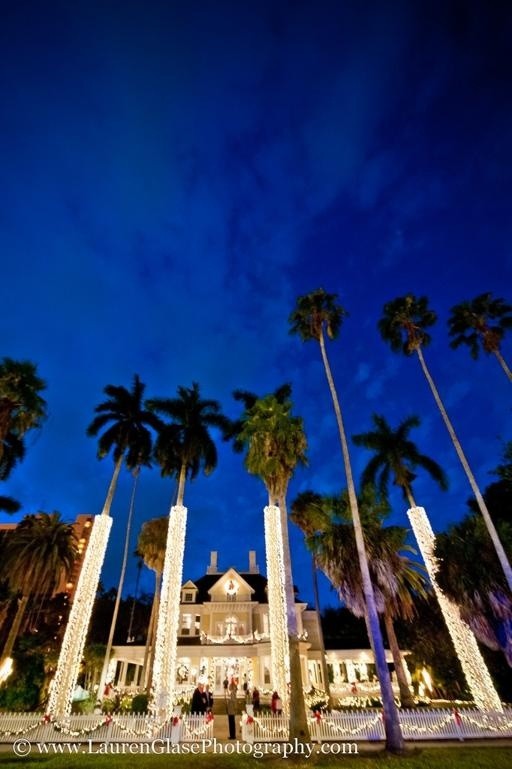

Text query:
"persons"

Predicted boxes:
[[189, 673, 283, 740]]
